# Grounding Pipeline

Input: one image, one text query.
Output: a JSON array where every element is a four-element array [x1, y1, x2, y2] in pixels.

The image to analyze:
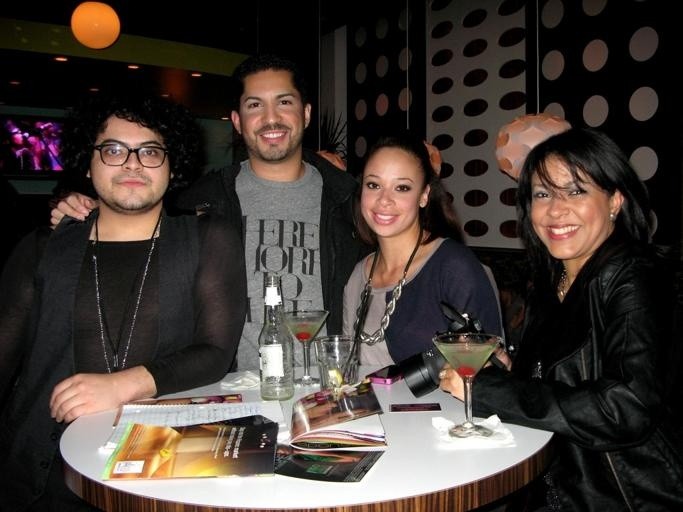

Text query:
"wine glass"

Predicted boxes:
[[432, 332, 500, 439], [282, 309, 329, 386]]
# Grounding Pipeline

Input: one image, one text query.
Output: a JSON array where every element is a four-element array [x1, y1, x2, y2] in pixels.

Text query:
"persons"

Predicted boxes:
[[343, 129, 504, 371], [0, 80, 247, 510], [50, 51, 442, 377], [437, 126, 680, 511]]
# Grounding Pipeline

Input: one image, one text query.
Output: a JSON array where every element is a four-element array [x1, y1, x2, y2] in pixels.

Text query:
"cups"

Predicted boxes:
[[315, 335, 361, 394]]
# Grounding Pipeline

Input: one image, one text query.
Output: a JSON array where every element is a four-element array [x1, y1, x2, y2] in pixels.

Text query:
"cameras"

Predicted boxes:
[[402, 302, 507, 398]]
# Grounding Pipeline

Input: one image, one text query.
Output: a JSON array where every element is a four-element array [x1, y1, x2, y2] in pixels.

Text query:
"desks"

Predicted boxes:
[[56, 360, 556, 510]]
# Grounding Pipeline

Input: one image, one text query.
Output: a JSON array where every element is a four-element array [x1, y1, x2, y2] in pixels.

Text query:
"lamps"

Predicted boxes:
[[300, 1, 348, 175], [492, 2, 579, 180], [387, 2, 446, 178]]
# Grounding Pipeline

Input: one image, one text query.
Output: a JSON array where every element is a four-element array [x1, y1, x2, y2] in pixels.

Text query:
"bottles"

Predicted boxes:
[[258, 272, 294, 403]]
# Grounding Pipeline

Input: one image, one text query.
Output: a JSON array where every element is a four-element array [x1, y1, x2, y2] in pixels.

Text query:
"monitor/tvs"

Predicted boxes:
[[0, 114, 68, 179]]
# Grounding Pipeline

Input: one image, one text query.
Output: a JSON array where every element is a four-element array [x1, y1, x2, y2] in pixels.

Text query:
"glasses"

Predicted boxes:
[[92, 144, 168, 168]]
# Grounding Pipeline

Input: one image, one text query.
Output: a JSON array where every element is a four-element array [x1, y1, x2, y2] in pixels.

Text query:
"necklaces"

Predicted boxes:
[[88, 208, 165, 375], [354, 226, 426, 344], [558, 268, 580, 299]]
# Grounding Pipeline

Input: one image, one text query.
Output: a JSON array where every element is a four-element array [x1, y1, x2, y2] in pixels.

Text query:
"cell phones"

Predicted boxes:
[[366, 365, 404, 385]]
